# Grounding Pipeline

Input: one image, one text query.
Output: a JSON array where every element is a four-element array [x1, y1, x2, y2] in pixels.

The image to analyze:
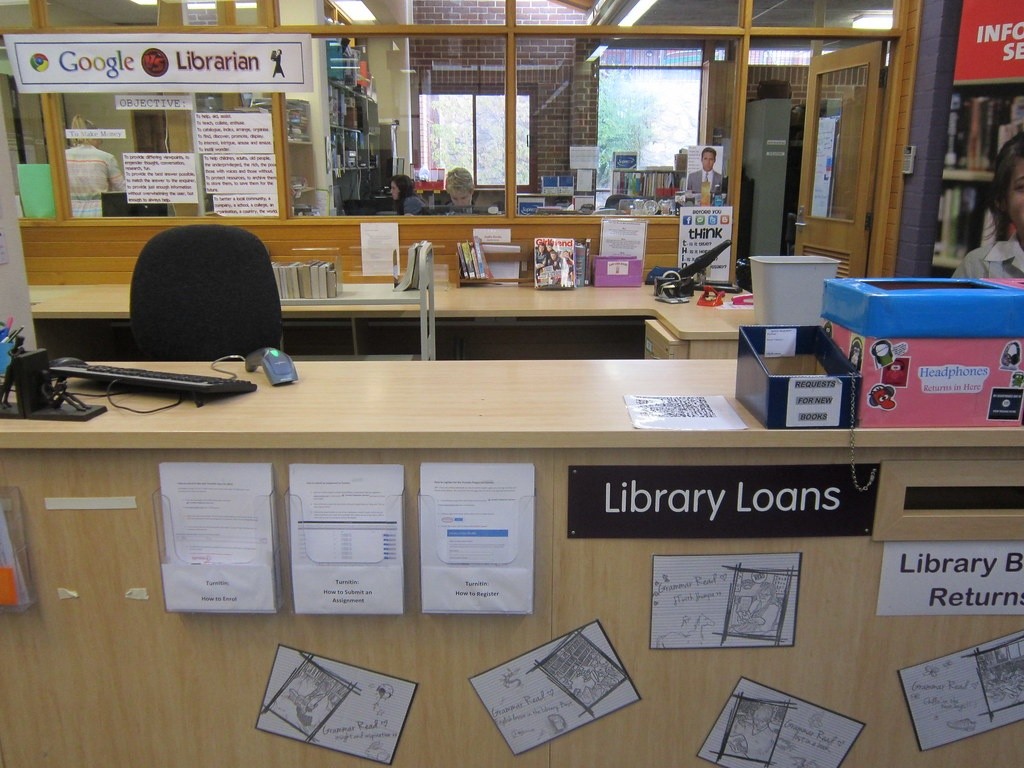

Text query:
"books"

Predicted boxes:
[[271, 236, 733, 299]]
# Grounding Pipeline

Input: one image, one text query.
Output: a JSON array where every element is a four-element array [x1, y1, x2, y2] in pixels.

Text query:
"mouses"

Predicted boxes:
[[48, 358, 88, 368]]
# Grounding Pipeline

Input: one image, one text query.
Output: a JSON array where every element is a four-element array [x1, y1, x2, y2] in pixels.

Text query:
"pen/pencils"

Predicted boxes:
[[0, 316, 24, 343]]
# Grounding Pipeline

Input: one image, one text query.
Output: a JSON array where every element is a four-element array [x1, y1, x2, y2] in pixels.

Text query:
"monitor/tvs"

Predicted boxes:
[[0, 104, 39, 353], [423, 205, 499, 215], [101, 192, 169, 217], [344, 197, 393, 216]]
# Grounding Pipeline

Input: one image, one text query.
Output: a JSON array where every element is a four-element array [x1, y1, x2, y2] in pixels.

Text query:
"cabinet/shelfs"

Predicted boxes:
[[267, 246, 437, 361], [931, 76, 1024, 272], [327, 76, 380, 208], [612, 169, 688, 202]]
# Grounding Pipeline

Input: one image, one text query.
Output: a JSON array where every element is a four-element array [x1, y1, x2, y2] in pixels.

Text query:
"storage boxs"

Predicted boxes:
[[518, 197, 545, 215], [735, 325, 861, 430], [102, 191, 169, 216], [591, 255, 645, 288], [613, 151, 637, 170], [822, 276, 1024, 428]]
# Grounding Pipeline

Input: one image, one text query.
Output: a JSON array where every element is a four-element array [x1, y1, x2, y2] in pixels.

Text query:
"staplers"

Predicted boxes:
[[731, 294, 754, 305]]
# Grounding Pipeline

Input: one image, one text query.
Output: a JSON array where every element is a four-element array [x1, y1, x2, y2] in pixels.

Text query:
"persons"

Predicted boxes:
[[688, 147, 723, 193], [390, 167, 474, 215], [950, 129, 1024, 280], [65, 114, 127, 219]]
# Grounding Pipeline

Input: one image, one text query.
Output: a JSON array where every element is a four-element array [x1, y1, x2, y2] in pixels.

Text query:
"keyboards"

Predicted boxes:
[[48, 363, 257, 408]]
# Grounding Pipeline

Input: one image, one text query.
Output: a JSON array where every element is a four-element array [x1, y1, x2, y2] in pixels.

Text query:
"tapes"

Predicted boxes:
[[662, 270, 681, 281]]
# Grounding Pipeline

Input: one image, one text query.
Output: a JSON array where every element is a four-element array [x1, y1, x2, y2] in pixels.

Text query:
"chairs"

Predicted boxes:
[[129, 223, 282, 362], [607, 194, 633, 210]]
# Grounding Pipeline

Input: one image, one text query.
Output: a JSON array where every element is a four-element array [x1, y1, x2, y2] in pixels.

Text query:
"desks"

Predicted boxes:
[[29, 278, 755, 362], [514, 191, 595, 216]]
